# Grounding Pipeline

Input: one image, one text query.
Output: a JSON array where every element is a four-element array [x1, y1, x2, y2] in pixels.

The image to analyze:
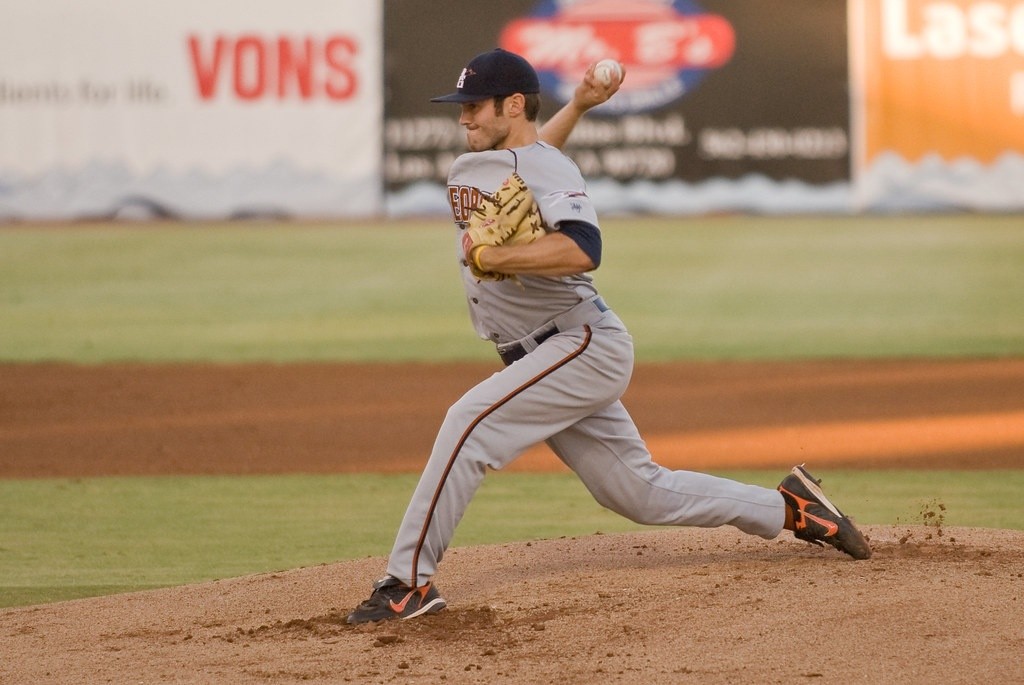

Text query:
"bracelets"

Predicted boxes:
[[475, 246, 491, 273]]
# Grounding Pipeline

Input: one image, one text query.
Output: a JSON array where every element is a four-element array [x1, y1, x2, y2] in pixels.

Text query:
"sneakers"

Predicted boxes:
[[346, 577, 446, 624], [777, 463, 873, 560]]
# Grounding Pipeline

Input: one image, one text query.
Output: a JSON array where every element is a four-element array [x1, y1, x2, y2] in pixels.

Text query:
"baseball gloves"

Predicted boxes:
[[461, 173, 550, 282]]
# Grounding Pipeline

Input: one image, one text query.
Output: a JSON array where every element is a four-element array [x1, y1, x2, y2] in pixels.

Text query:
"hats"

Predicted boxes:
[[430, 49, 540, 102]]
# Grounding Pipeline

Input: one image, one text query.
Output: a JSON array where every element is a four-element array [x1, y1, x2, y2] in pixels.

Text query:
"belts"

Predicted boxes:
[[499, 297, 610, 367]]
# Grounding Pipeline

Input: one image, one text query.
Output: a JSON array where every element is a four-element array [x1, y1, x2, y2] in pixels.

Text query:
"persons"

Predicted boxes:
[[347, 46, 871, 622]]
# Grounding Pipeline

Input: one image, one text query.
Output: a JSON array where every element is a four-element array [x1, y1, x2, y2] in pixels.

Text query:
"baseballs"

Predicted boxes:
[[593, 60, 625, 87]]
